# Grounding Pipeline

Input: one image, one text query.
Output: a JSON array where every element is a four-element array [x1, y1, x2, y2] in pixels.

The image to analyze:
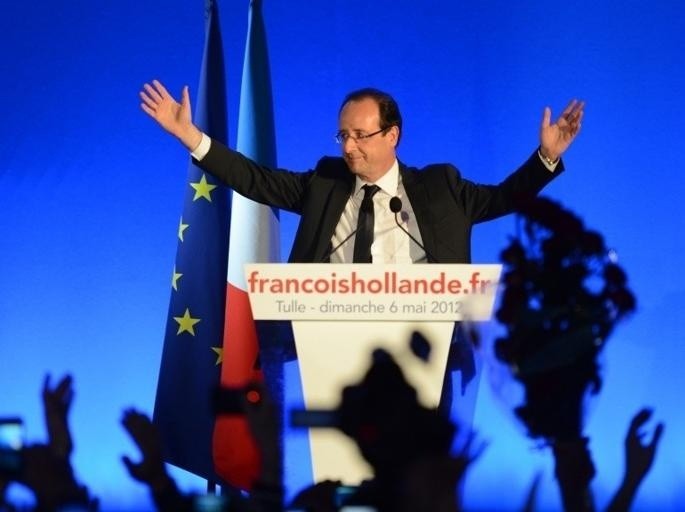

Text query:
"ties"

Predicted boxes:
[[352, 181, 381, 262]]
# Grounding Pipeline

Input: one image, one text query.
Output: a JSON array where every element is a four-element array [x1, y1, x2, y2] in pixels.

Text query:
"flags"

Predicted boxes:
[[145, 0, 228, 487], [209, 1, 296, 499]]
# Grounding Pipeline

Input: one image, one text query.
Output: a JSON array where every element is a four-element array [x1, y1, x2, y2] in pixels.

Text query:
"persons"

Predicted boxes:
[[139, 76, 585, 415]]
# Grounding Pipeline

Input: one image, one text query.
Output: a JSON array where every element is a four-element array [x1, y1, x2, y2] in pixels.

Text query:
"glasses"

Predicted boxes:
[[334, 127, 389, 144]]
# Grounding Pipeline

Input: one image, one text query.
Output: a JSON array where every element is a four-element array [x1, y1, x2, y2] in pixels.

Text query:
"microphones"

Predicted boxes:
[[320, 199, 375, 263], [390, 196, 439, 265]]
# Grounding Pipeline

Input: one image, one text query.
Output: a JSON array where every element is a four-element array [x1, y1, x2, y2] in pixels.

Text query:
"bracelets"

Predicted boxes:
[[539, 147, 558, 165]]
